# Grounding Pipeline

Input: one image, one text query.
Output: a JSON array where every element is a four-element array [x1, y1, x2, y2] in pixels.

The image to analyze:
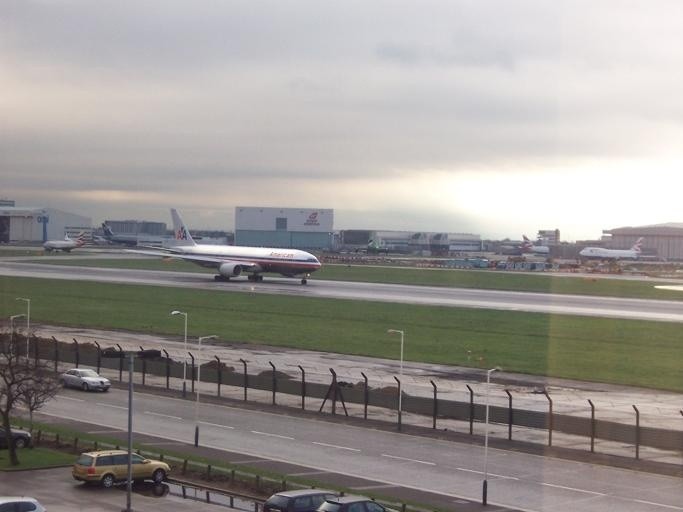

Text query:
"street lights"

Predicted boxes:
[[387, 329, 403, 430], [101, 346, 161, 510], [195, 335, 219, 447], [171, 311, 187, 397], [10, 297, 30, 359], [483, 366, 503, 505]]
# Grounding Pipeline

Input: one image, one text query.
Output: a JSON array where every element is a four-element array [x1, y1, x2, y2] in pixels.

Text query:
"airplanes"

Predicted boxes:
[[169, 207, 321, 284], [579, 237, 645, 261], [517, 234, 550, 257], [93, 222, 138, 247], [43, 232, 85, 253]]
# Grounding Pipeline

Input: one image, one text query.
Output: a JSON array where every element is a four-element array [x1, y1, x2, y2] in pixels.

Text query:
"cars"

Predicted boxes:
[[57, 368, 111, 392], [0, 496, 47, 512], [263, 489, 400, 512], [0, 426, 32, 448], [72, 450, 171, 488]]
